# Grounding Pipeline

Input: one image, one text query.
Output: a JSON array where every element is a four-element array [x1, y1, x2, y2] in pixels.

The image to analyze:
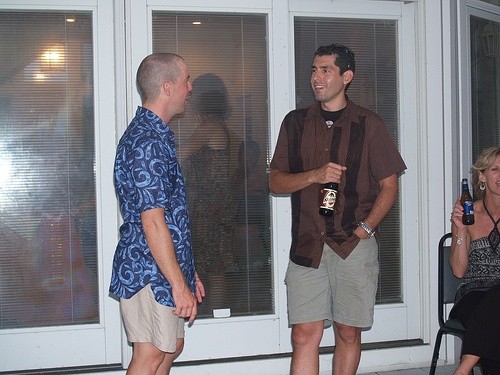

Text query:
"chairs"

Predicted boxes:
[[429, 233, 474, 375]]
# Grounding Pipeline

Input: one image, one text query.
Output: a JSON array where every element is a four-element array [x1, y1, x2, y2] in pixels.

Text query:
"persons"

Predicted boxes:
[[449, 145, 500, 375], [269, 44, 407, 375], [109, 54, 205, 375], [234, 142, 266, 272], [179, 73, 231, 314]]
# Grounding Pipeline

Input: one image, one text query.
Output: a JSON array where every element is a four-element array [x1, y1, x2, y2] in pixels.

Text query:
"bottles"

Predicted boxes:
[[462, 178, 474, 225], [319, 182, 339, 216]]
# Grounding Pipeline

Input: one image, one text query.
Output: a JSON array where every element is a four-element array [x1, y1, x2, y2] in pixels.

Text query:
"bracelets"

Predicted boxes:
[[359, 222, 375, 238], [456, 235, 466, 244]]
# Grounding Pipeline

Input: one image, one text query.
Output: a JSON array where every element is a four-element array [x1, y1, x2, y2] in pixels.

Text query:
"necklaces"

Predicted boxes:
[[482, 198, 500, 251]]
